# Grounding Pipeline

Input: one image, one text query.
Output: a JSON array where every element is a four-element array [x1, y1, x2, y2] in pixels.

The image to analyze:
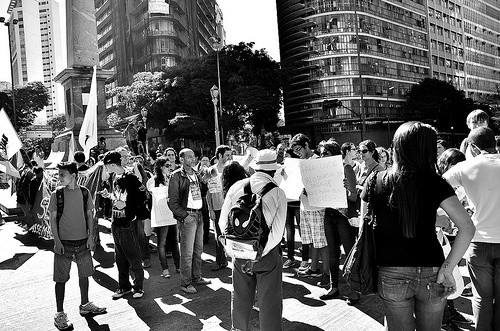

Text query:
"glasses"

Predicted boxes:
[[293, 144, 305, 154], [357, 148, 372, 154], [351, 147, 357, 151], [163, 164, 171, 168], [320, 151, 332, 157]]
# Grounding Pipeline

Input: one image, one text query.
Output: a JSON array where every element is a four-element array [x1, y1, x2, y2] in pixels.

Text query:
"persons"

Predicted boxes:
[[167, 148, 211, 293], [0, 109, 500, 331], [281, 133, 329, 287], [49, 163, 107, 331]]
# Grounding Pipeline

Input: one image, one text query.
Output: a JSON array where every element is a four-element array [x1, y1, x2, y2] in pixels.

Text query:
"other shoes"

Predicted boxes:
[[346, 291, 360, 304], [317, 276, 329, 286], [297, 268, 323, 277], [283, 258, 296, 268], [141, 259, 229, 294], [112, 288, 131, 297], [133, 289, 144, 297], [319, 289, 340, 300], [299, 261, 310, 271]]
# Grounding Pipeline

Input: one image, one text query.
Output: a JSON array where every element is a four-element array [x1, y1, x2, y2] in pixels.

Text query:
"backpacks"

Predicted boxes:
[[224, 177, 279, 257], [122, 175, 150, 220]]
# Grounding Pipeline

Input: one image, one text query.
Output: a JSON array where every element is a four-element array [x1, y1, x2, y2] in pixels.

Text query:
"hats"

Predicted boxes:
[[250, 148, 282, 170], [104, 152, 121, 164]]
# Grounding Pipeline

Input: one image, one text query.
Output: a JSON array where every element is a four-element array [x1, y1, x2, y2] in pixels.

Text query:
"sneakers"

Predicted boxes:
[[54, 311, 73, 330], [80, 301, 106, 313]]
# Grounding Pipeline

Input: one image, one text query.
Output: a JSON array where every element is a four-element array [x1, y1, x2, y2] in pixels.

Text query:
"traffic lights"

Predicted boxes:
[[322, 99, 342, 112]]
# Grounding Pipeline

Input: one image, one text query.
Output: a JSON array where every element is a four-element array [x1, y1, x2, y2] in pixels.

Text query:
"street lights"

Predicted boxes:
[[210, 85, 220, 148], [0, 17, 19, 133], [209, 35, 224, 144]]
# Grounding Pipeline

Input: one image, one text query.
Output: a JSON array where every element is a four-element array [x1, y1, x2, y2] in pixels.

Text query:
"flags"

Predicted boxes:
[[78, 65, 97, 162], [0, 107, 23, 160]]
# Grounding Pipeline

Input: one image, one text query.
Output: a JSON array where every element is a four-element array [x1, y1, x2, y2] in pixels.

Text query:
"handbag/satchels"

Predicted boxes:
[[437, 227, 464, 300], [341, 172, 379, 295], [151, 186, 177, 228]]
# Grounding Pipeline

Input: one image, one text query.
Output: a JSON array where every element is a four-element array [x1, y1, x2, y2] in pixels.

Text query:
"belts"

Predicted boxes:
[[185, 208, 201, 212]]
